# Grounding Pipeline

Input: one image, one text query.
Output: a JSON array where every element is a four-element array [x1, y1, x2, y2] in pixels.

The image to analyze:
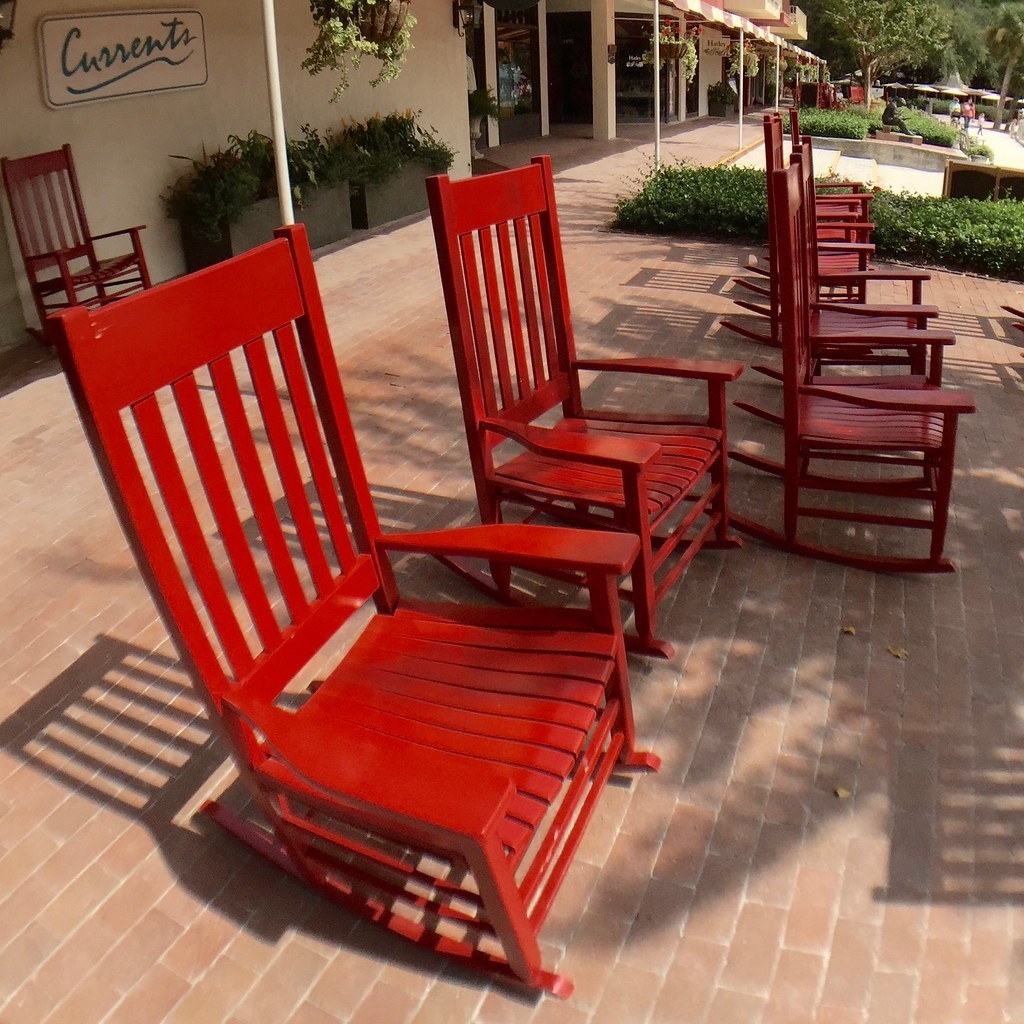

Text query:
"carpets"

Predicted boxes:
[[471, 156, 511, 177]]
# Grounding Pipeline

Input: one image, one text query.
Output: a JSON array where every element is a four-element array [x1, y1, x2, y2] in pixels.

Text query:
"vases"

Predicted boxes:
[[660, 43, 687, 59]]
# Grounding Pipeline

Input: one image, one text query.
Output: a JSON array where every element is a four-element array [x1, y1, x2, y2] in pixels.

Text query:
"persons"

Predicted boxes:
[[961, 98, 975, 129], [949, 98, 961, 122], [883, 98, 914, 135], [978, 113, 985, 135]]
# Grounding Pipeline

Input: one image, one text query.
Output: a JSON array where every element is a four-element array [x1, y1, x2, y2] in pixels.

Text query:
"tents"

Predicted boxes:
[[981, 94, 1013, 109]]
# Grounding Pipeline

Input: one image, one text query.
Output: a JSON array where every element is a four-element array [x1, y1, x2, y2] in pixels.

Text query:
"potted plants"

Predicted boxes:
[[708, 79, 738, 117], [765, 53, 818, 83], [161, 125, 461, 261], [299, 0, 418, 104], [468, 84, 503, 158]]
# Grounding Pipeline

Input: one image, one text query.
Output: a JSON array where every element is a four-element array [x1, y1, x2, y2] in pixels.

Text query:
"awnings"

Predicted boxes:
[[654, 0, 827, 171]]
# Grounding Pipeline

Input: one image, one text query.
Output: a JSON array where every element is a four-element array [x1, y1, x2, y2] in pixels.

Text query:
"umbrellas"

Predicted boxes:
[[912, 86, 939, 96], [941, 90, 968, 100], [883, 82, 908, 96]]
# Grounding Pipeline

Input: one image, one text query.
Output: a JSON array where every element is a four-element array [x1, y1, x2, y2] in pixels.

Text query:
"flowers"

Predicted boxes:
[[726, 38, 761, 78], [341, 106, 419, 153], [642, 13, 704, 83]]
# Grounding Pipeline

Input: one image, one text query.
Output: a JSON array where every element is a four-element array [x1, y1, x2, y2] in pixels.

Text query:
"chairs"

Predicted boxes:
[[762, 106, 876, 272], [425, 155, 742, 659], [734, 112, 870, 303], [703, 154, 977, 574], [0, 143, 153, 348], [719, 118, 873, 358], [45, 222, 662, 1001], [731, 135, 956, 442]]
